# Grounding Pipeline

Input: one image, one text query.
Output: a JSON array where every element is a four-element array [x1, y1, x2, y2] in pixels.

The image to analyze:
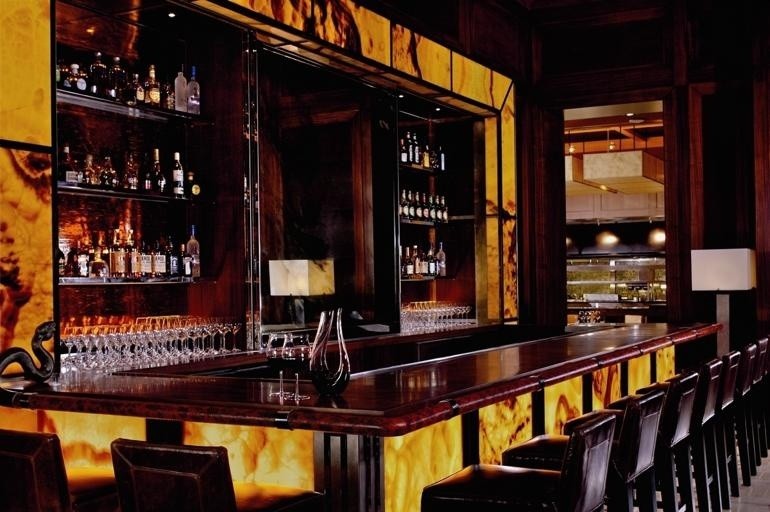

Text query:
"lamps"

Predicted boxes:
[[0, 428, 118, 512], [690, 246, 756, 358], [564, 124, 665, 196], [268, 257, 336, 327], [110, 436, 324, 512], [617, 305, 650, 325]]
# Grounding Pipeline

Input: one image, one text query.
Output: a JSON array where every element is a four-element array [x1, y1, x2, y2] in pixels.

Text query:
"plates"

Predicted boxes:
[[564, 195, 665, 224], [399, 161, 438, 283], [565, 257, 667, 304], [55, 88, 215, 287]]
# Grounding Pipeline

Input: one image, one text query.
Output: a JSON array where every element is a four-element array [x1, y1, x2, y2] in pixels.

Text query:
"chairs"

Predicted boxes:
[[617, 305, 650, 325], [421, 410, 618, 512], [0, 428, 118, 512], [502, 339, 770, 512], [110, 436, 324, 512]]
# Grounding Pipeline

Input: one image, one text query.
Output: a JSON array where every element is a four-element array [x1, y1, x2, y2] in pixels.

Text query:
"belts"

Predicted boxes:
[[266, 332, 313, 403], [401, 299, 473, 334], [49, 313, 241, 372], [54, 374, 217, 392]]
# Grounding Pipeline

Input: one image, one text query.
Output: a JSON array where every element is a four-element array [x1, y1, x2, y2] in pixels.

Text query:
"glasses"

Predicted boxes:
[[564, 124, 665, 196], [268, 257, 336, 327], [690, 246, 756, 358]]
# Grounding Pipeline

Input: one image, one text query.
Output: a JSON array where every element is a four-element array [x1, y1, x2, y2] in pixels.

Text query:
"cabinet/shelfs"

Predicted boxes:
[[565, 257, 667, 304], [400, 129, 447, 171], [564, 195, 665, 224], [399, 189, 449, 223], [399, 161, 438, 283], [632, 287, 640, 302], [56, 220, 204, 283], [400, 241, 448, 281], [578, 311, 601, 323], [59, 143, 202, 199], [56, 49, 202, 115], [55, 88, 215, 287]]
